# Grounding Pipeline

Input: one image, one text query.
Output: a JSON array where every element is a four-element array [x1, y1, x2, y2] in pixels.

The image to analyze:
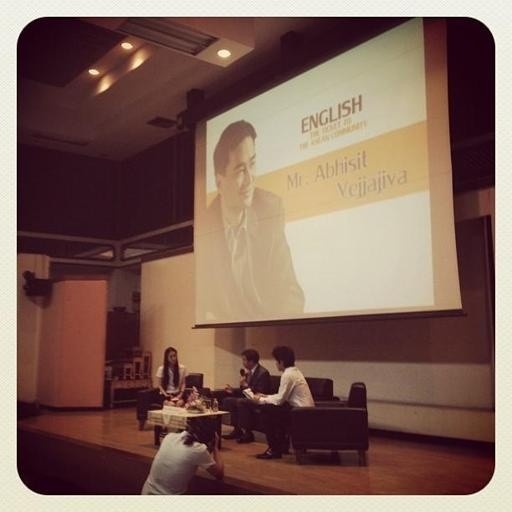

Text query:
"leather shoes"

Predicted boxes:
[[256, 445, 282, 458], [237, 430, 255, 443], [221, 427, 244, 440]]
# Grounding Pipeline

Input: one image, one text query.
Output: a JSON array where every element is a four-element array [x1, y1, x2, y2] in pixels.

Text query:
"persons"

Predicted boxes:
[[248, 345, 316, 460], [156, 346, 187, 439], [199, 116, 308, 321], [141, 416, 227, 495], [220, 347, 272, 444]]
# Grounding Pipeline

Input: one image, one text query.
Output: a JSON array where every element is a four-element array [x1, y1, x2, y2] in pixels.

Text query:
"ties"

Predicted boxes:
[[246, 369, 252, 384]]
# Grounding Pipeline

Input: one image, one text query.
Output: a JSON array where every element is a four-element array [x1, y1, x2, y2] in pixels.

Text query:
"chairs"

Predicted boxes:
[[135, 373, 370, 467]]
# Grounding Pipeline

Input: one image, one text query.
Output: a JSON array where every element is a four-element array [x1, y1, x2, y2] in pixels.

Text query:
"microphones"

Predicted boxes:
[[240, 368, 245, 377]]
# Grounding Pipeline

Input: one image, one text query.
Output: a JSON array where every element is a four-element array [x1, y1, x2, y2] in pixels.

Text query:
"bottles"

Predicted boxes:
[[213, 398, 218, 412]]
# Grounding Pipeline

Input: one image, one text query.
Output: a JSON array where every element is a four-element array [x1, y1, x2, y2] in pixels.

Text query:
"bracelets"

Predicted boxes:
[[180, 391, 185, 393]]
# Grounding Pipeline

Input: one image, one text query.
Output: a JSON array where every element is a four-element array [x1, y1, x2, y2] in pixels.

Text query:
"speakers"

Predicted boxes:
[[25, 279, 49, 296]]
